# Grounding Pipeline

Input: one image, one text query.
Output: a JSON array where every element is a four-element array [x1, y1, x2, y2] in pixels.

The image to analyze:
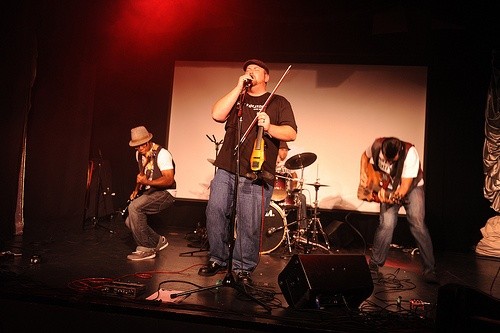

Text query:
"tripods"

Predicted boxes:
[[170, 84, 274, 312], [295, 186, 332, 254]]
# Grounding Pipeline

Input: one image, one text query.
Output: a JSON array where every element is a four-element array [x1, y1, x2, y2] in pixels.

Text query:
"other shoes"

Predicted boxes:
[[424, 271, 439, 284], [368, 262, 378, 274]]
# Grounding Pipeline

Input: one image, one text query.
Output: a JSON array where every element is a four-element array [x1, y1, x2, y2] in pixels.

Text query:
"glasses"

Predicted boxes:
[[135, 143, 147, 150]]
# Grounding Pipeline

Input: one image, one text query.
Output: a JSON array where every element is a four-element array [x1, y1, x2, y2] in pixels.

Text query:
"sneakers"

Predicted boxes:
[[157, 235, 168, 251], [127, 248, 155, 261]]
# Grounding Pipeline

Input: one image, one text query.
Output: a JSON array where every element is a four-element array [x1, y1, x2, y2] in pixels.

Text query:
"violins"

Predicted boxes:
[[249, 105, 269, 174]]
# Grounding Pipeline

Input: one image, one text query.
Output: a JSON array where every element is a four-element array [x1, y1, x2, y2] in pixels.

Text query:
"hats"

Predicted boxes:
[[243, 59, 269, 75], [129, 125, 153, 147]]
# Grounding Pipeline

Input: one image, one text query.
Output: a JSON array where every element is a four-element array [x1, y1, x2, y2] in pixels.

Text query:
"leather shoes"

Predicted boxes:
[[238, 270, 252, 285], [197, 261, 227, 276]]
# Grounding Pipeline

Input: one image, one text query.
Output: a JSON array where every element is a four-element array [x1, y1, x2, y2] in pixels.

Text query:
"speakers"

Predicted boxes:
[[323, 220, 365, 250], [278, 253, 375, 310]]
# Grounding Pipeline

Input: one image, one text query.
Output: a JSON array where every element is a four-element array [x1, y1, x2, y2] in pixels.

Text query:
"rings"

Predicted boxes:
[[263, 119, 265, 123]]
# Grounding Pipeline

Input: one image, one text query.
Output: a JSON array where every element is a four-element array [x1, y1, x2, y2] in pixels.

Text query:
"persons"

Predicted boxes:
[[271, 142, 309, 230], [198, 59, 298, 286], [125, 126, 177, 261], [360, 137, 443, 284]]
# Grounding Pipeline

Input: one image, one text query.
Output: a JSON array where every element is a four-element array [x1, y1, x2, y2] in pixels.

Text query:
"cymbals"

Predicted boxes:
[[306, 183, 330, 187], [207, 158, 217, 166], [284, 152, 317, 170]]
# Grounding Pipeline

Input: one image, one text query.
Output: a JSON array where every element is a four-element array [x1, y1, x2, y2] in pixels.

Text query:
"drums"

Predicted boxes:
[[233, 200, 288, 255], [270, 176, 288, 202]]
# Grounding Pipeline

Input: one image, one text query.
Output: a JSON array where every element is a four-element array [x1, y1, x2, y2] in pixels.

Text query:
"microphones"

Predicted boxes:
[[244, 79, 252, 84], [267, 226, 275, 236]]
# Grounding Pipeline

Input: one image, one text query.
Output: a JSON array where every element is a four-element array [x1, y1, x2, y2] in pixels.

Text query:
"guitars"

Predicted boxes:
[[120, 148, 158, 219], [357, 153, 405, 205]]
